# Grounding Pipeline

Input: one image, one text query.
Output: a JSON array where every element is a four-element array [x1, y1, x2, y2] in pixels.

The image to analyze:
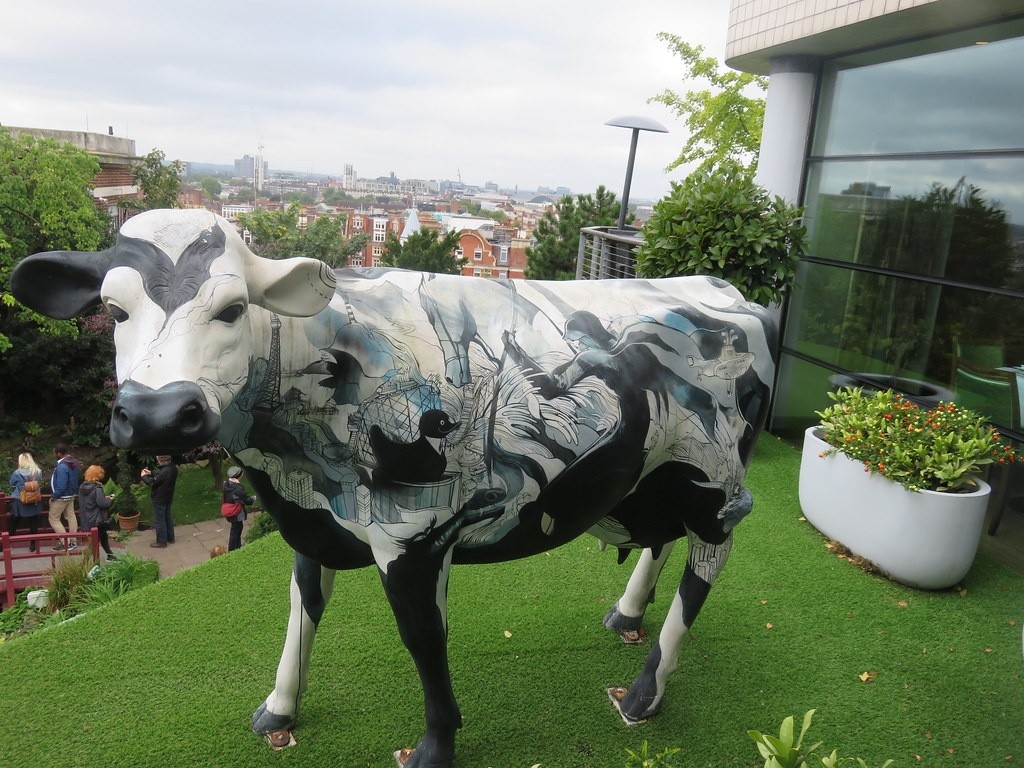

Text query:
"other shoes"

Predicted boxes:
[[167, 537, 175, 544], [29, 544, 35, 552], [106, 555, 117, 562], [151, 543, 167, 548], [51, 543, 77, 552]]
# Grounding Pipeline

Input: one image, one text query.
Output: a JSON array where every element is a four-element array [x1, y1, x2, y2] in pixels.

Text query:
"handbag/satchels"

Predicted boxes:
[[103, 518, 116, 530], [221, 502, 242, 518]]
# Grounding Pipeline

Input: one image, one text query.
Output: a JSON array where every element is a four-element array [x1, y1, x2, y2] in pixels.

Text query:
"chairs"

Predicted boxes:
[[949, 325, 1023, 534]]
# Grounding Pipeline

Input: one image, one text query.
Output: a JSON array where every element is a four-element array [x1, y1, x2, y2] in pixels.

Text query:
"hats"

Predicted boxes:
[[227, 466, 242, 478]]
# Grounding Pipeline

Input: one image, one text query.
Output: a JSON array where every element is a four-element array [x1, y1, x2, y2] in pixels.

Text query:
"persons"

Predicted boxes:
[[0, 453, 42, 552], [141, 455, 178, 548], [222, 466, 257, 552], [78, 465, 117, 562], [48, 444, 83, 552]]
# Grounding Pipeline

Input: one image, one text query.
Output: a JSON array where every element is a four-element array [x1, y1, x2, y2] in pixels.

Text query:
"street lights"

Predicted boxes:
[[603, 113, 670, 231]]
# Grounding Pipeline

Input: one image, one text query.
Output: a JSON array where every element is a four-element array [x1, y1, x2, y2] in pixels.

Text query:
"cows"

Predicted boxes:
[[10, 207, 781, 768]]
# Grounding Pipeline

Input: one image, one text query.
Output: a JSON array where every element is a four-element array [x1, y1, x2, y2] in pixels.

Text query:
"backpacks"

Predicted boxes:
[[17, 469, 41, 506]]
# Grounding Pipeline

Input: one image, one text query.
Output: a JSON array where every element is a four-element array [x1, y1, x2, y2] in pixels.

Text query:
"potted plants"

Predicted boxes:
[[110, 448, 142, 531], [798, 385, 1022, 589]]
